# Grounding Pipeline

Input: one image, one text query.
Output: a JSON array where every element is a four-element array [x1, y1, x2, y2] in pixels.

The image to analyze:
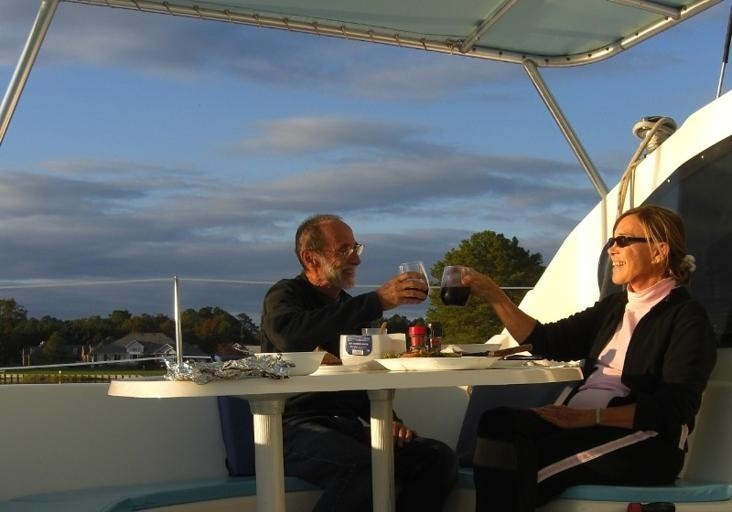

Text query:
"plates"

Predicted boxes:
[[492, 359, 531, 369], [452, 344, 502, 354], [373, 355, 502, 371]]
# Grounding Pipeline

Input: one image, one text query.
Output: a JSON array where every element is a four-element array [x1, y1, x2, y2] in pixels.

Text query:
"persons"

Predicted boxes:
[[233, 211, 458, 510], [460, 204, 720, 510]]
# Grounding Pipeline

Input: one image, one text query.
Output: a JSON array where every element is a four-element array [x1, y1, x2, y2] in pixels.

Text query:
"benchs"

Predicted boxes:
[[1, 378, 731, 512]]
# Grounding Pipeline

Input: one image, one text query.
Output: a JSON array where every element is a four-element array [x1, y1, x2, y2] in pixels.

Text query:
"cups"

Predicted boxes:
[[339, 334, 385, 371], [399, 261, 430, 302], [439, 265, 472, 307], [362, 327, 388, 360], [387, 333, 407, 358]]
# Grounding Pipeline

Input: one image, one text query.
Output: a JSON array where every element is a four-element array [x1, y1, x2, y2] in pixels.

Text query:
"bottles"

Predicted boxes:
[[408, 324, 427, 354], [426, 321, 443, 351]]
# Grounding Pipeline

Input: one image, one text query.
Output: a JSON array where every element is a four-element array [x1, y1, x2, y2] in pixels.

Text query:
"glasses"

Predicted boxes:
[[607, 235, 654, 248], [320, 243, 366, 257]]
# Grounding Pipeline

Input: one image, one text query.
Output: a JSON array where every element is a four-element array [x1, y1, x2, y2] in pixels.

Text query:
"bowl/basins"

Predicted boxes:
[[252, 352, 328, 376]]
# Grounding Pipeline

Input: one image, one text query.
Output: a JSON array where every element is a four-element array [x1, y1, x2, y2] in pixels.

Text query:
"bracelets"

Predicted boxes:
[[595, 406, 601, 425]]
